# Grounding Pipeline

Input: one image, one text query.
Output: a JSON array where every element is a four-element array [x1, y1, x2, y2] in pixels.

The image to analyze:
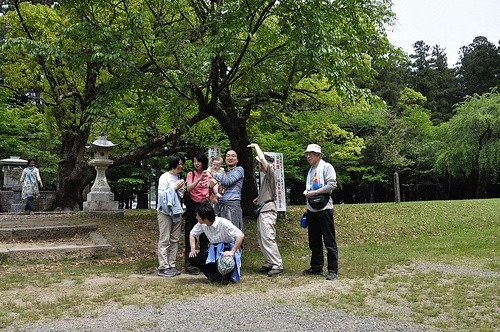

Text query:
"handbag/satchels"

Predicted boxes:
[[182, 170, 195, 217], [216, 252, 235, 275], [253, 203, 263, 220], [308, 193, 330, 210]]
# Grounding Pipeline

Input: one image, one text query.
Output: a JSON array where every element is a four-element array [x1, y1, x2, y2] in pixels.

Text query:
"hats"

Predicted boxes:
[[303, 144, 325, 159], [256, 154, 275, 166]]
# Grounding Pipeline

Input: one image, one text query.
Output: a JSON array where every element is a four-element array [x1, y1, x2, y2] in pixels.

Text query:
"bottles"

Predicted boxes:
[[301, 212, 308, 228]]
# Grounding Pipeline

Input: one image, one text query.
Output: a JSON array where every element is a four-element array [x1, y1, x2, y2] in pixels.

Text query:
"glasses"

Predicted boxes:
[[178, 163, 186, 167], [256, 161, 262, 165]]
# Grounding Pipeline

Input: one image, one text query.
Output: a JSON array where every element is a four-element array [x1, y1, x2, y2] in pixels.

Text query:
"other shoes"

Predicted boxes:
[[203, 274, 221, 283], [158, 268, 181, 277], [257, 266, 272, 273], [220, 275, 229, 284], [326, 272, 337, 280], [267, 269, 283, 276], [303, 266, 323, 275], [185, 268, 200, 275]]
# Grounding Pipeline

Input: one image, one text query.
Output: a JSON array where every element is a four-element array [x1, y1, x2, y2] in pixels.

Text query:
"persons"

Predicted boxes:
[[247, 143, 283, 276], [188, 203, 245, 285], [156, 154, 185, 277], [207, 148, 244, 233], [183, 153, 212, 275], [302, 144, 338, 280], [19, 158, 43, 212]]
